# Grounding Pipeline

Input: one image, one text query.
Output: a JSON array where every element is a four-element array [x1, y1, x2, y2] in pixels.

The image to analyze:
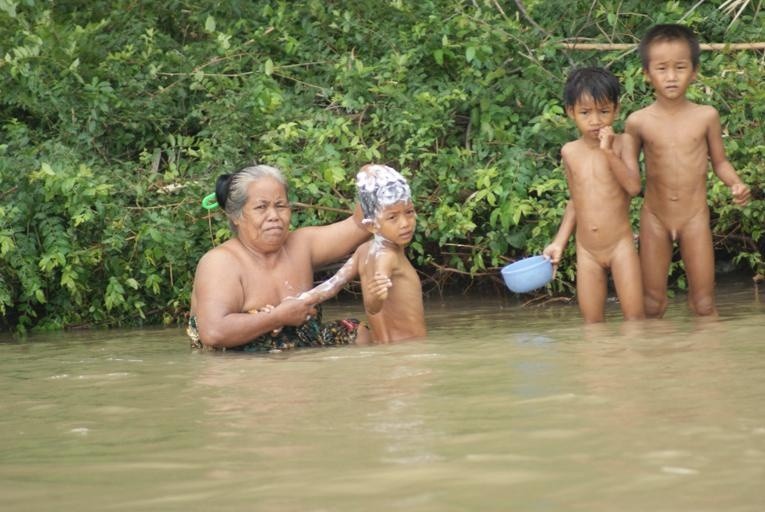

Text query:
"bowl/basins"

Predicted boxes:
[[500, 255, 556, 294]]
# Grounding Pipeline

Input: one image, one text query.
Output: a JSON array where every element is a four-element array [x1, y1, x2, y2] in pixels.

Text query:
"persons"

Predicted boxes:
[[542, 66, 646, 325], [257, 165, 428, 344], [185, 164, 373, 355], [622, 24, 753, 319]]
